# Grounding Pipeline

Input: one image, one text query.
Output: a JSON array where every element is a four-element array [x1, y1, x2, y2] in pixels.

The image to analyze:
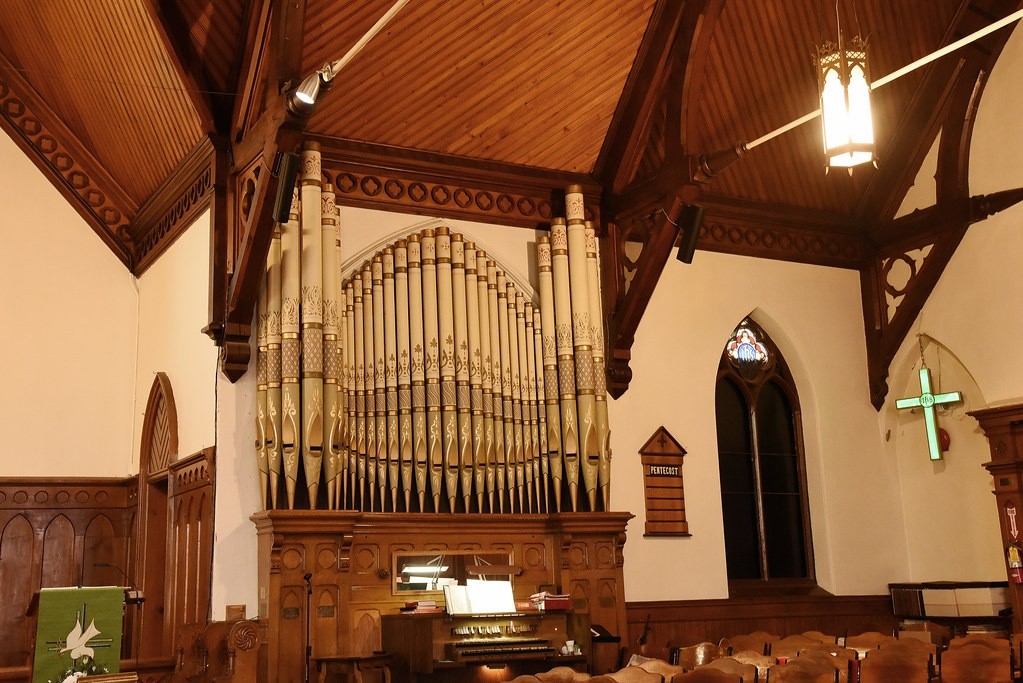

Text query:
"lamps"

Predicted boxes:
[[272, 152, 299, 223], [811, 0, 879, 176], [676, 204, 704, 264], [466, 554, 522, 581], [401, 548, 450, 591], [296, 63, 336, 104]]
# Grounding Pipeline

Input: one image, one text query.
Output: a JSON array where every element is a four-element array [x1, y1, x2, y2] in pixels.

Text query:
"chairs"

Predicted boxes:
[[500, 623, 1023, 683]]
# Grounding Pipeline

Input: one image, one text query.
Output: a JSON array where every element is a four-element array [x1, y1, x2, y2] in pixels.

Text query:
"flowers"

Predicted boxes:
[[48, 653, 109, 683]]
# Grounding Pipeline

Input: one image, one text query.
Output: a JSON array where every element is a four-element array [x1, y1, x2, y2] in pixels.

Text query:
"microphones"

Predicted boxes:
[[93, 563, 146, 605]]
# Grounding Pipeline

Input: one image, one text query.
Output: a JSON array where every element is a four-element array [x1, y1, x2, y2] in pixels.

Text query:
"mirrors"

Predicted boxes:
[[392, 549, 515, 595]]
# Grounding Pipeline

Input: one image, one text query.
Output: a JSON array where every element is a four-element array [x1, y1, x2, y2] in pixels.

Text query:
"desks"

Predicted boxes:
[[311, 653, 396, 683]]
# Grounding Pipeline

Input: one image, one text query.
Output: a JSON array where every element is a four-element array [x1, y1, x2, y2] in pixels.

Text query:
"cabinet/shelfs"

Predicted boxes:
[[380, 609, 593, 683]]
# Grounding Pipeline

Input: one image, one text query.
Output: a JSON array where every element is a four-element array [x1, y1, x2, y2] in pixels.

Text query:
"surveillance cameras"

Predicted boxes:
[[272, 151, 300, 223], [676, 204, 704, 264]]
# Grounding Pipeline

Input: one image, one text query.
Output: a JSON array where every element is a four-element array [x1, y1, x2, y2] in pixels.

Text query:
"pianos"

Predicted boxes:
[[379, 607, 593, 683]]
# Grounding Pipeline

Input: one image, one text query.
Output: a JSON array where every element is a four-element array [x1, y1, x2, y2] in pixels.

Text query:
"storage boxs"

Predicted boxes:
[[533, 600, 571, 610]]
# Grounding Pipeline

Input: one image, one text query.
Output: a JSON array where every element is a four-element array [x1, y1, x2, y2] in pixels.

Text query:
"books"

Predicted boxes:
[[529, 591, 570, 600], [127, 590, 144, 598], [400, 601, 442, 614]]
